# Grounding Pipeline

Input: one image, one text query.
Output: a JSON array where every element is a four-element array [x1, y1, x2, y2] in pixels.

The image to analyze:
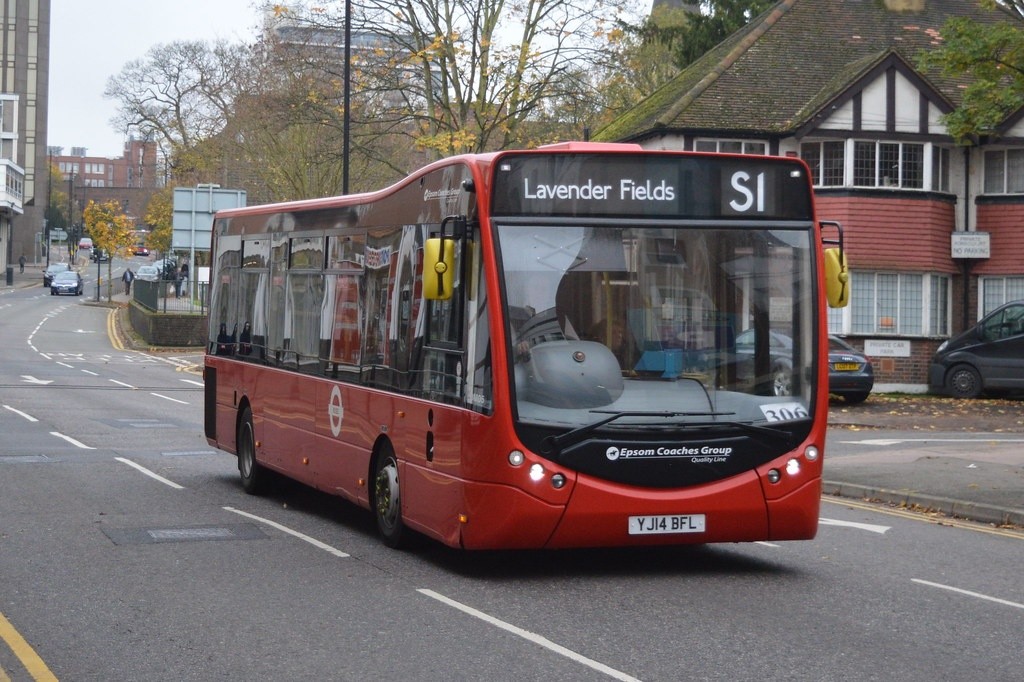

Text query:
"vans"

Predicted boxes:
[[927, 298, 1024, 398]]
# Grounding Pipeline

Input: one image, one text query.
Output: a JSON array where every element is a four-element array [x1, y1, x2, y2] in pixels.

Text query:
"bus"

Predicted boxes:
[[203, 141, 850, 555], [127, 230, 150, 256]]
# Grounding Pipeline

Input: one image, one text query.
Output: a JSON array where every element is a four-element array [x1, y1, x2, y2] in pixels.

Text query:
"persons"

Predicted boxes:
[[173, 267, 184, 298], [121, 267, 134, 296], [19, 253, 27, 274], [510, 274, 581, 355]]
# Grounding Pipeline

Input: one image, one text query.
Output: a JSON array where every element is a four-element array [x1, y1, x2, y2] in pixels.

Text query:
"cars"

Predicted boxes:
[[696, 325, 875, 404], [93, 249, 109, 263], [50, 271, 84, 296], [89, 247, 94, 259], [134, 256, 177, 289], [42, 263, 73, 287], [78, 237, 92, 249]]
